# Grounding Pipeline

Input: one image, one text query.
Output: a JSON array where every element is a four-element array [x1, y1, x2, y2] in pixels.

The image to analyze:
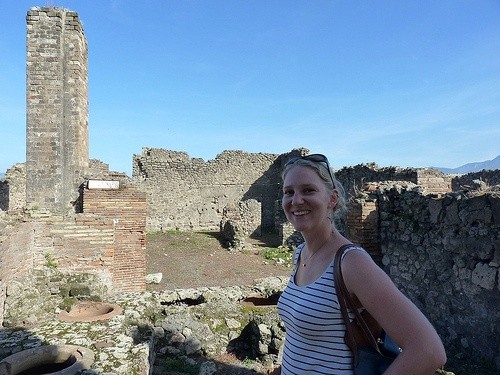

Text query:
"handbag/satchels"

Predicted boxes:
[[333, 244, 402, 375]]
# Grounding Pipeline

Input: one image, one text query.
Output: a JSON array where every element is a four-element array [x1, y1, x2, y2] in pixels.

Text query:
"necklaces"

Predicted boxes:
[[302, 231, 335, 267]]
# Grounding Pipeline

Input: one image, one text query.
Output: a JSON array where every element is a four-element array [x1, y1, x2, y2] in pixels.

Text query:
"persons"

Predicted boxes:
[[277, 154, 447, 375]]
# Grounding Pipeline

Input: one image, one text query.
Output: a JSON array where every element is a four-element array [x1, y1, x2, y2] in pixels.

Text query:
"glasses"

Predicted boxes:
[[283, 153, 336, 191]]
